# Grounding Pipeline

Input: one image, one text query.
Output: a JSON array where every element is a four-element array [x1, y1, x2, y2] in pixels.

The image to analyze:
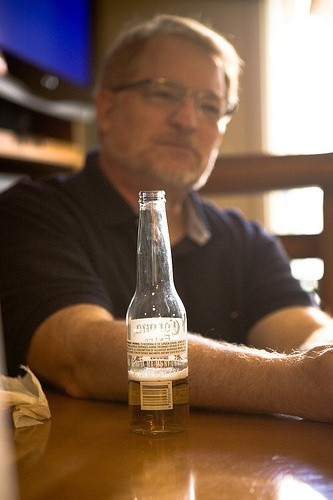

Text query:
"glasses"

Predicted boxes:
[[113, 79, 236, 126]]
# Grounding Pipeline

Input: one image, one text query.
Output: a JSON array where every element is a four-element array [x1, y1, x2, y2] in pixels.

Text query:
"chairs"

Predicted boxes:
[[85, 153, 333, 319]]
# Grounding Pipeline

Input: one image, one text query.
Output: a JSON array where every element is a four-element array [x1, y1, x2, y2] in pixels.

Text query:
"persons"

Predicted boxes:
[[0, 15, 333, 423]]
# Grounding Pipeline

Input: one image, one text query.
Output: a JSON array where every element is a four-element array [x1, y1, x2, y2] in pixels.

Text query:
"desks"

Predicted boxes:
[[16, 392, 333, 500]]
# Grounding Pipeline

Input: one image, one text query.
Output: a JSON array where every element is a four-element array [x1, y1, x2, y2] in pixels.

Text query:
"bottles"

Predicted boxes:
[[126, 189, 194, 436]]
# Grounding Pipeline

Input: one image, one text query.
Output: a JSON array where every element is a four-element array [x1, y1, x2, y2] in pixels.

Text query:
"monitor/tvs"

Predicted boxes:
[[0, 0, 98, 97]]
